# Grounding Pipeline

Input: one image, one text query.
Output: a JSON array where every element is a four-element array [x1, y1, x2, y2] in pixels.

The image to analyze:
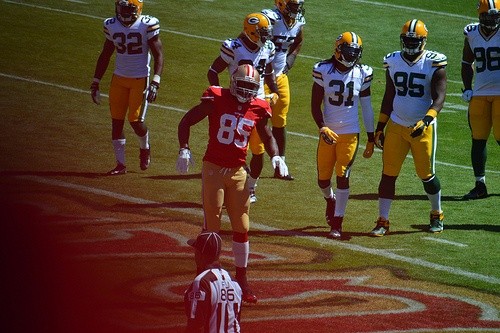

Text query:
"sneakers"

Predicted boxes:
[[107, 160, 127, 177], [188, 228, 206, 247], [369, 216, 390, 237], [428, 210, 444, 234], [462, 184, 488, 200], [139, 143, 151, 170], [242, 290, 257, 304]]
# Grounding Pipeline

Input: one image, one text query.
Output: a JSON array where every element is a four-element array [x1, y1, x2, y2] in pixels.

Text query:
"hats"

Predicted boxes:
[[187, 231, 222, 255]]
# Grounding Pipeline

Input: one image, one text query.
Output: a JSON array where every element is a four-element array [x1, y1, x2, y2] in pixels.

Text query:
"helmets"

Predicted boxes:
[[274, 0, 306, 19], [244, 12, 272, 47], [115, 0, 144, 25], [477, 0, 500, 27], [335, 31, 363, 67], [230, 64, 260, 99], [400, 19, 428, 55]]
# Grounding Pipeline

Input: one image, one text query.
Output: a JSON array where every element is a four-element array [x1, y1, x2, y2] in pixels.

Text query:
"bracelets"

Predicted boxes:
[[93, 78, 100, 84], [152, 74, 161, 84]]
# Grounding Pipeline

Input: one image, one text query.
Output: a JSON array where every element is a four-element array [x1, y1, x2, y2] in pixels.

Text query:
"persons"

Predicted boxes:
[[369, 20, 448, 237], [184, 231, 243, 333], [460, 0, 500, 199], [261, 0, 307, 179], [176, 63, 289, 303], [311, 31, 375, 238], [207, 13, 279, 209], [90, 0, 164, 175]]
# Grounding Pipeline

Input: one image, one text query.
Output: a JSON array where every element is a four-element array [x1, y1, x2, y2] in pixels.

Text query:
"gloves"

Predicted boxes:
[[375, 129, 384, 150], [462, 89, 473, 102], [143, 83, 157, 103], [363, 142, 375, 157], [90, 81, 101, 105], [269, 155, 288, 177], [175, 148, 195, 173], [320, 127, 338, 146], [408, 119, 429, 138], [283, 62, 290, 76]]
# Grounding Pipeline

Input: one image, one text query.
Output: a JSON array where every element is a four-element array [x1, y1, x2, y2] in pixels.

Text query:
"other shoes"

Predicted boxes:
[[248, 186, 257, 204], [274, 166, 295, 181], [324, 193, 336, 226], [329, 216, 344, 237]]
